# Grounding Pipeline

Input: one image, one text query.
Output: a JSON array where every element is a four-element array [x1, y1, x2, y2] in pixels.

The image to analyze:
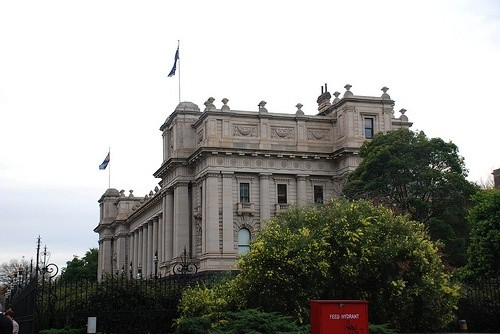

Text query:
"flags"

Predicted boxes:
[[167, 45, 181, 76], [98, 152, 110, 170]]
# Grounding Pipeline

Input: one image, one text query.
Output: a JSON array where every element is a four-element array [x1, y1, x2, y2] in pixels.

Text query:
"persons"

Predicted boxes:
[[5, 308, 19, 334], [0, 304, 14, 334]]
